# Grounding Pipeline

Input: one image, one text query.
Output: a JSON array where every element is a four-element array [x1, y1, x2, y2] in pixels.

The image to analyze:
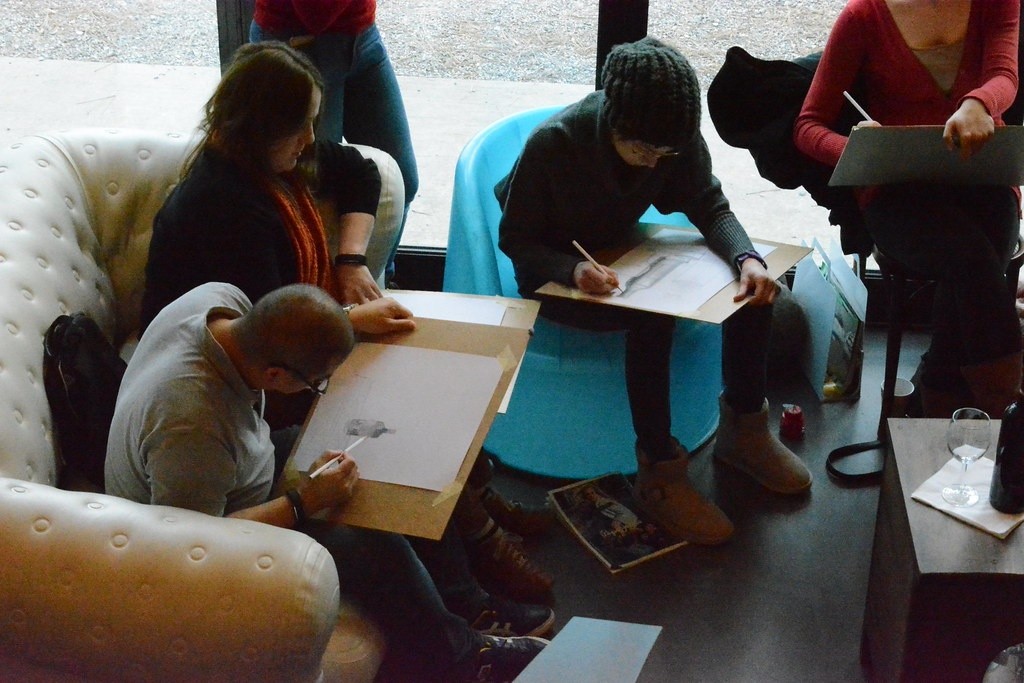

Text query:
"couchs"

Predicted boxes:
[[0, 126, 407, 683]]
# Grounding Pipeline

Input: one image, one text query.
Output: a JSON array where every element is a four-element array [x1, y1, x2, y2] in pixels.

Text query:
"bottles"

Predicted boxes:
[[991, 382, 1024, 514]]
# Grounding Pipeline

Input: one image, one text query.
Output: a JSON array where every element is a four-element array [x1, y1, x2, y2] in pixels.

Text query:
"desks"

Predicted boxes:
[[859, 417, 1024, 683]]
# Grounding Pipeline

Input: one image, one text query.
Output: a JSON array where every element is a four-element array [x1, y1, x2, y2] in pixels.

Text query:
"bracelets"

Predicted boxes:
[[736, 251, 768, 274], [343, 303, 357, 312], [287, 489, 308, 527], [335, 255, 368, 267]]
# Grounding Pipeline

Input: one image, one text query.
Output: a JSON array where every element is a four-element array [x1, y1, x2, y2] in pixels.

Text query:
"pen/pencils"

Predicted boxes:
[[309, 435, 368, 480], [843, 90, 874, 122], [572, 239, 624, 294]]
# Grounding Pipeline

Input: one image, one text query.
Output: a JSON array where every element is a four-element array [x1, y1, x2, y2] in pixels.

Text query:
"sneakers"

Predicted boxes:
[[466, 596, 556, 641], [445, 632, 554, 683]]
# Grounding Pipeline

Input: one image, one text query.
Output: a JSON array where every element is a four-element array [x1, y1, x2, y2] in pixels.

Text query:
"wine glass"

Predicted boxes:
[[941, 407, 992, 507]]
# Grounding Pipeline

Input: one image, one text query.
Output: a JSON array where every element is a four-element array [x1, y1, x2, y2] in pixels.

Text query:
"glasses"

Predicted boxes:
[[269, 359, 332, 399]]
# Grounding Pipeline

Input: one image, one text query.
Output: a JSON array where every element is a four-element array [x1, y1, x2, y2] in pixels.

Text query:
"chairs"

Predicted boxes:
[[439, 104, 732, 479], [800, 44, 1024, 483]]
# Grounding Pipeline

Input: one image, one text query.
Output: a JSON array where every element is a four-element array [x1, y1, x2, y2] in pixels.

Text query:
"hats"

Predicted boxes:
[[598, 36, 706, 151]]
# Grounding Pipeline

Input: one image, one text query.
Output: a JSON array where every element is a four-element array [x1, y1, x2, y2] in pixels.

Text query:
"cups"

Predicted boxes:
[[882, 377, 915, 418]]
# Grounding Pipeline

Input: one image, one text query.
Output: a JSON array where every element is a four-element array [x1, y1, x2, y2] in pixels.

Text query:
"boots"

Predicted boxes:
[[475, 537, 555, 595], [713, 394, 813, 496], [627, 437, 736, 546], [484, 494, 557, 543]]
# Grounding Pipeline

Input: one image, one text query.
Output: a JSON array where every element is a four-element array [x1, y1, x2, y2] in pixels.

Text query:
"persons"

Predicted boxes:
[[139, 40, 561, 603], [248, 1, 420, 290], [795, 1, 1024, 419], [102, 279, 553, 683], [495, 37, 817, 547]]
